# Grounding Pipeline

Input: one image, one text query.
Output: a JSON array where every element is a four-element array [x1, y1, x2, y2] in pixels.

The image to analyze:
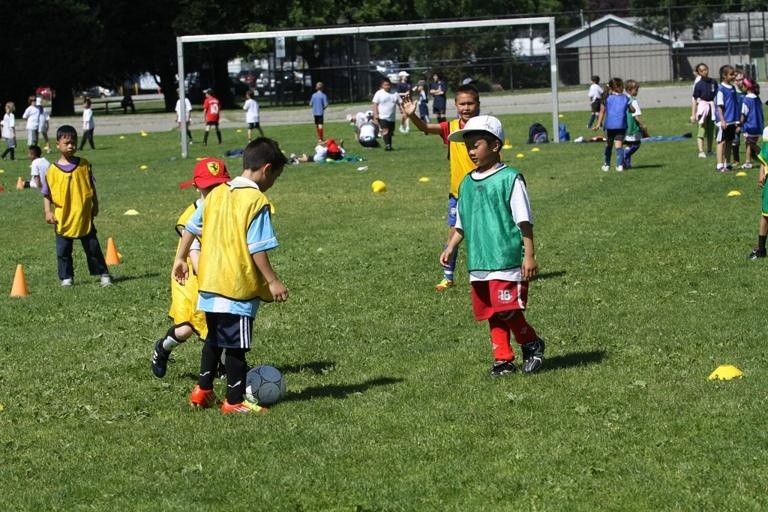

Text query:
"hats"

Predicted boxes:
[[448, 115, 505, 146], [192, 159, 232, 189], [398, 71, 411, 76]]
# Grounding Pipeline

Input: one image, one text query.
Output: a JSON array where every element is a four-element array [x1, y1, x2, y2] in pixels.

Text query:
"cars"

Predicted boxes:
[[80, 84, 117, 99]]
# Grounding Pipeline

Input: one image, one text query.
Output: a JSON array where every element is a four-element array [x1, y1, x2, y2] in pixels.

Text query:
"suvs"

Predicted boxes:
[[177, 56, 413, 106]]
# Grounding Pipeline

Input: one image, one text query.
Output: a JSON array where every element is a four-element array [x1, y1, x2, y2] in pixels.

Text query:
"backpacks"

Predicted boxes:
[[527, 123, 548, 142]]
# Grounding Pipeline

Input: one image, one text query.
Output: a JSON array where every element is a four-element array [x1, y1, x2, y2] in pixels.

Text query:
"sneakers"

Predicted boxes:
[[601, 165, 624, 171], [749, 247, 766, 260], [60, 277, 75, 287], [434, 278, 454, 291], [99, 274, 115, 287], [521, 336, 545, 373], [188, 384, 222, 407], [152, 336, 171, 379], [716, 164, 754, 172], [220, 398, 270, 416], [488, 363, 517, 375], [697, 152, 706, 158], [381, 119, 415, 150]]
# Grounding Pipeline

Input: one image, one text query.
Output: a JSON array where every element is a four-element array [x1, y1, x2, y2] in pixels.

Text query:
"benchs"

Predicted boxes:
[[91, 99, 128, 114]]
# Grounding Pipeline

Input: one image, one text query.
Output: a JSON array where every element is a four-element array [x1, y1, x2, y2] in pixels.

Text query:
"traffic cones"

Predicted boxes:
[[102, 233, 124, 266], [6, 262, 30, 299], [14, 175, 26, 192]]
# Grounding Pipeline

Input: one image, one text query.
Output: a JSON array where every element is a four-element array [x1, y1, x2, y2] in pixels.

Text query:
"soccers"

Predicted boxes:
[[245, 366, 286, 407]]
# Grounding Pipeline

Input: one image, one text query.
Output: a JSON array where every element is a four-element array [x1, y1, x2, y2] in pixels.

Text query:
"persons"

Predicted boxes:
[[34, 104, 52, 153], [0, 101, 18, 161], [345, 67, 448, 152], [308, 81, 329, 141], [149, 156, 232, 381], [296, 136, 346, 164], [399, 84, 481, 293], [172, 87, 194, 146], [201, 87, 224, 146], [746, 125, 768, 259], [75, 98, 96, 151], [21, 94, 41, 147], [241, 90, 266, 143], [121, 93, 137, 114], [41, 123, 114, 289], [437, 114, 546, 375], [586, 63, 766, 174], [173, 137, 289, 416], [15, 144, 52, 190]]
